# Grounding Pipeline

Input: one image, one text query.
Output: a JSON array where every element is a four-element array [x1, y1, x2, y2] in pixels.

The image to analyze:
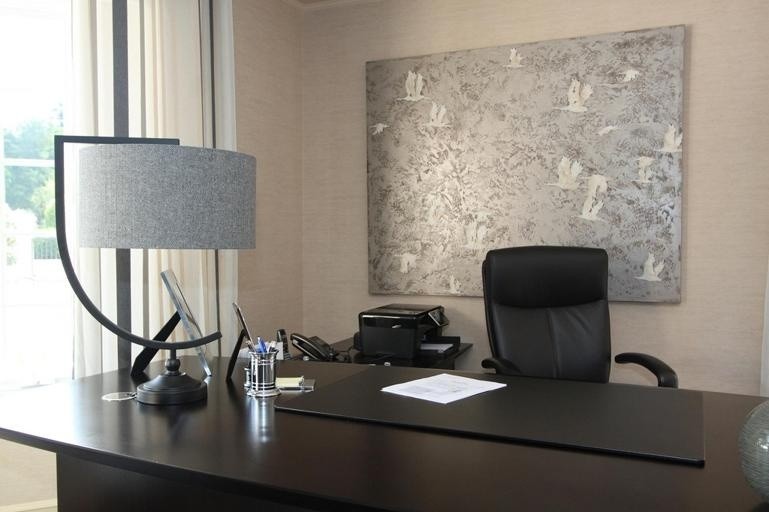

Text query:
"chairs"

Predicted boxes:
[[481, 245, 679, 388]]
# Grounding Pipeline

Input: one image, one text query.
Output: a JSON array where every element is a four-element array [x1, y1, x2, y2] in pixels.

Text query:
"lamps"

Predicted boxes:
[[53, 134, 257, 407]]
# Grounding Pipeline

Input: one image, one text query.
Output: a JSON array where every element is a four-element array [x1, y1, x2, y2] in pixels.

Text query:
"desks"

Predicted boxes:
[[1, 354, 769, 512], [287, 335, 474, 369]]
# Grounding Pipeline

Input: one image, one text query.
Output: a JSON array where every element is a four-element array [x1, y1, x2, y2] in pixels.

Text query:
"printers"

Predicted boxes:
[[359, 303, 461, 364]]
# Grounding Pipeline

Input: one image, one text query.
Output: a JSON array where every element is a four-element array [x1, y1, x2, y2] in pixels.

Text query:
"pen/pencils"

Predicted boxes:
[[246, 336, 279, 352]]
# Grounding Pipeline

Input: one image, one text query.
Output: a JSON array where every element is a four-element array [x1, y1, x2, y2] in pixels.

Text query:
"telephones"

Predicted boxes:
[[290, 332, 339, 361]]
